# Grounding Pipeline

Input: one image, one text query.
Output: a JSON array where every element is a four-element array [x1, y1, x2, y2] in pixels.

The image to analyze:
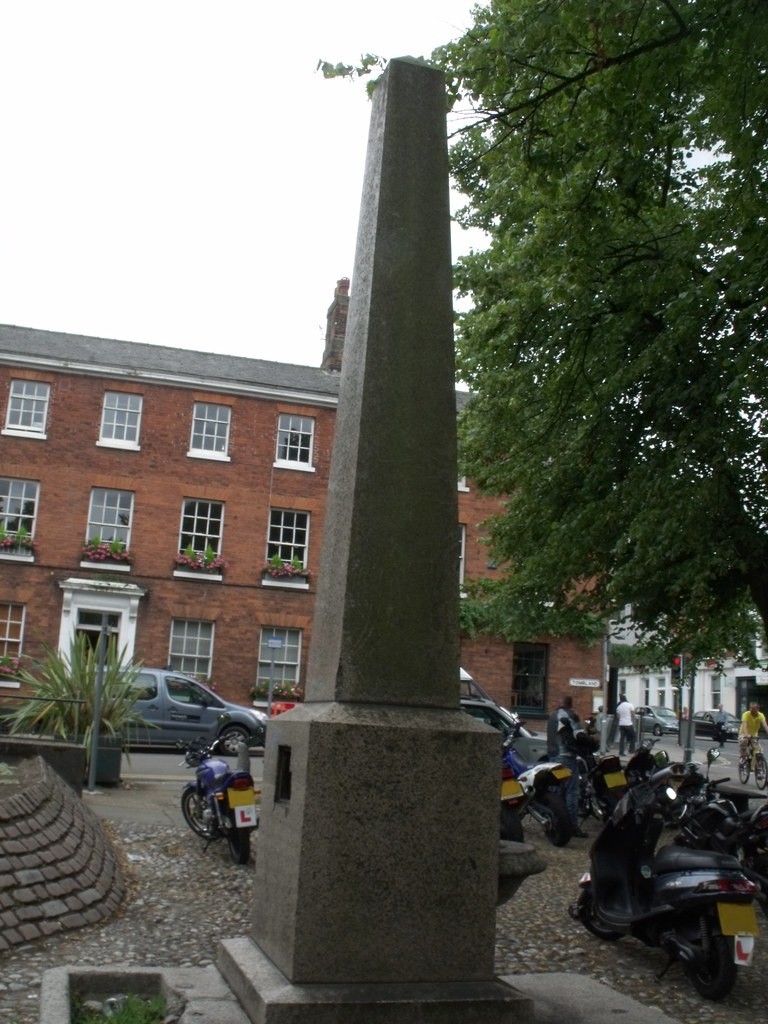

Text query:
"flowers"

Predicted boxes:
[[248, 680, 305, 701], [80, 543, 135, 563], [173, 553, 228, 574], [0, 657, 21, 675], [1, 534, 40, 551], [168, 674, 218, 692], [257, 561, 314, 579]]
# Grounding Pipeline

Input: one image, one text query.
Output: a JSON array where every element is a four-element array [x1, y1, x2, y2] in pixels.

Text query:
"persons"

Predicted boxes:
[[616, 696, 637, 757], [682, 706, 689, 720], [594, 705, 611, 752], [713, 704, 727, 736], [551, 696, 580, 752], [739, 702, 768, 768]]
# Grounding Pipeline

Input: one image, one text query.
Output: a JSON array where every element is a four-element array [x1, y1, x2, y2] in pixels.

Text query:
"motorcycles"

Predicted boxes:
[[495, 712, 577, 848], [497, 752, 535, 842], [578, 758, 758, 1002], [569, 720, 630, 821], [174, 712, 259, 865], [629, 736, 711, 819], [672, 771, 767, 920]]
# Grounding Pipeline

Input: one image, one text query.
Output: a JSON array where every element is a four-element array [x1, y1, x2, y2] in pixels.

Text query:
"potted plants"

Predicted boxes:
[[0, 624, 162, 788]]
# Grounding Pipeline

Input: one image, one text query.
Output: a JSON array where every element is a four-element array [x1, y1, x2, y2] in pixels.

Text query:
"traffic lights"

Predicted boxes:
[[672, 654, 681, 686]]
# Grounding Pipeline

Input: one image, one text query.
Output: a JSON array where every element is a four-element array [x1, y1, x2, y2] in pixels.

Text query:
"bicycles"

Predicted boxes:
[[737, 735, 768, 792]]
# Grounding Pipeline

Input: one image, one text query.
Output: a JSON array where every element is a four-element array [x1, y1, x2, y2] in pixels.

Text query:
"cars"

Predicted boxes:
[[97, 662, 270, 756], [462, 700, 550, 764], [635, 705, 681, 736], [692, 708, 742, 740]]
